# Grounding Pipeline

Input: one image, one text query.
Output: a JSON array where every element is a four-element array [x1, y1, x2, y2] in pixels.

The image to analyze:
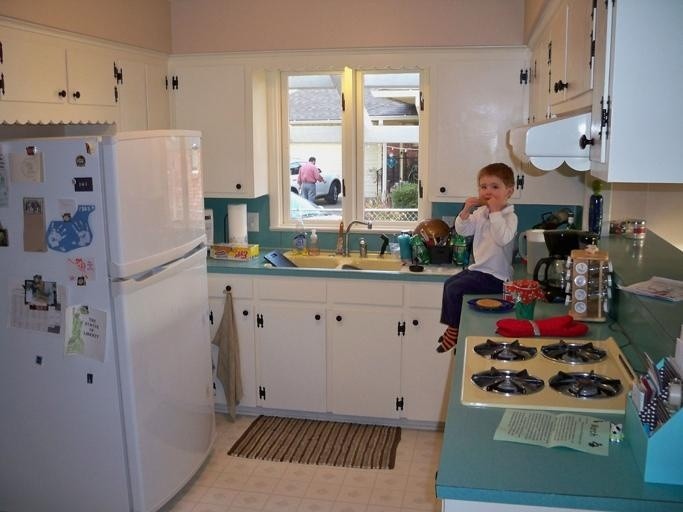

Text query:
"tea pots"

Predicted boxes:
[[518, 229, 550, 275]]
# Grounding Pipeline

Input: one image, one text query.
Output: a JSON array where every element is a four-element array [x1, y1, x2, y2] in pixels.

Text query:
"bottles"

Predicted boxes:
[[398, 231, 411, 259], [566, 211, 577, 231], [410, 232, 467, 265], [624, 218, 646, 239]]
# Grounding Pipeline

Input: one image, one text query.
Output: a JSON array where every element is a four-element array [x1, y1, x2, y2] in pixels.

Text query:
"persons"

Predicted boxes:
[[297, 156, 321, 202], [435, 163, 519, 353]]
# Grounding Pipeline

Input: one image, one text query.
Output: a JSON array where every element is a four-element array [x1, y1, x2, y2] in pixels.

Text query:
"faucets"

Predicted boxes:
[[343, 220, 373, 258]]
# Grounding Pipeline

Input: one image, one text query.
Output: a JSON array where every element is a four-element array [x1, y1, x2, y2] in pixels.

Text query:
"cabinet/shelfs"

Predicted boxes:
[[167, 50, 269, 199], [0, 13, 116, 127], [115, 40, 170, 133], [528, 1, 683, 184]]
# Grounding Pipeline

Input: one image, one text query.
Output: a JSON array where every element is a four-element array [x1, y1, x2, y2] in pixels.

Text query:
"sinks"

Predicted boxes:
[[264, 250, 343, 273], [338, 254, 404, 273]]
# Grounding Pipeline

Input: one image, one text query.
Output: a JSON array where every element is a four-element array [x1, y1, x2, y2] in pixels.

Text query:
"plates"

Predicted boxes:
[[467, 297, 515, 313]]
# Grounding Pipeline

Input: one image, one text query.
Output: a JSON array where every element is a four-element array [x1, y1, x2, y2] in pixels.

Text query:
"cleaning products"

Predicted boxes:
[[308, 229, 321, 256]]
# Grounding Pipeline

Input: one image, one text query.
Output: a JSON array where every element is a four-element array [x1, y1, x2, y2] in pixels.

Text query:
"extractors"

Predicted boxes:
[[509, 113, 592, 173]]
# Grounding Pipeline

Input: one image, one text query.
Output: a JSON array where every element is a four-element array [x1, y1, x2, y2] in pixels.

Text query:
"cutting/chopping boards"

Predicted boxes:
[[263, 249, 297, 267]]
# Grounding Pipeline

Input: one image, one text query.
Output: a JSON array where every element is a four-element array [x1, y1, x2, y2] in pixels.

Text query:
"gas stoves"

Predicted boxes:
[[461, 336, 639, 415]]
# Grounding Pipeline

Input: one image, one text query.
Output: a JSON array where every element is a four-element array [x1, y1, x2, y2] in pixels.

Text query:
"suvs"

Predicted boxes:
[[289, 160, 340, 203]]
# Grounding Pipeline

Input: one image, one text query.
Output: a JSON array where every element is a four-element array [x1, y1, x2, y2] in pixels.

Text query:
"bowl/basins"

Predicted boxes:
[[412, 219, 450, 239]]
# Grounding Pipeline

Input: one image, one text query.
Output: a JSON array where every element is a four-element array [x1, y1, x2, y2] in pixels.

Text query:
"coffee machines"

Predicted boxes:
[[533, 231, 598, 303]]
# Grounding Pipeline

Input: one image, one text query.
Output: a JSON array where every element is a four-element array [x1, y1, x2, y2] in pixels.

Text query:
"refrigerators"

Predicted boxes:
[[1, 129, 218, 512]]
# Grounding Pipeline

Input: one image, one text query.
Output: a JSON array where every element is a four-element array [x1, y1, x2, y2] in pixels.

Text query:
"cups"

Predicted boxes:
[[514, 280, 540, 320], [565, 258, 613, 319]]
